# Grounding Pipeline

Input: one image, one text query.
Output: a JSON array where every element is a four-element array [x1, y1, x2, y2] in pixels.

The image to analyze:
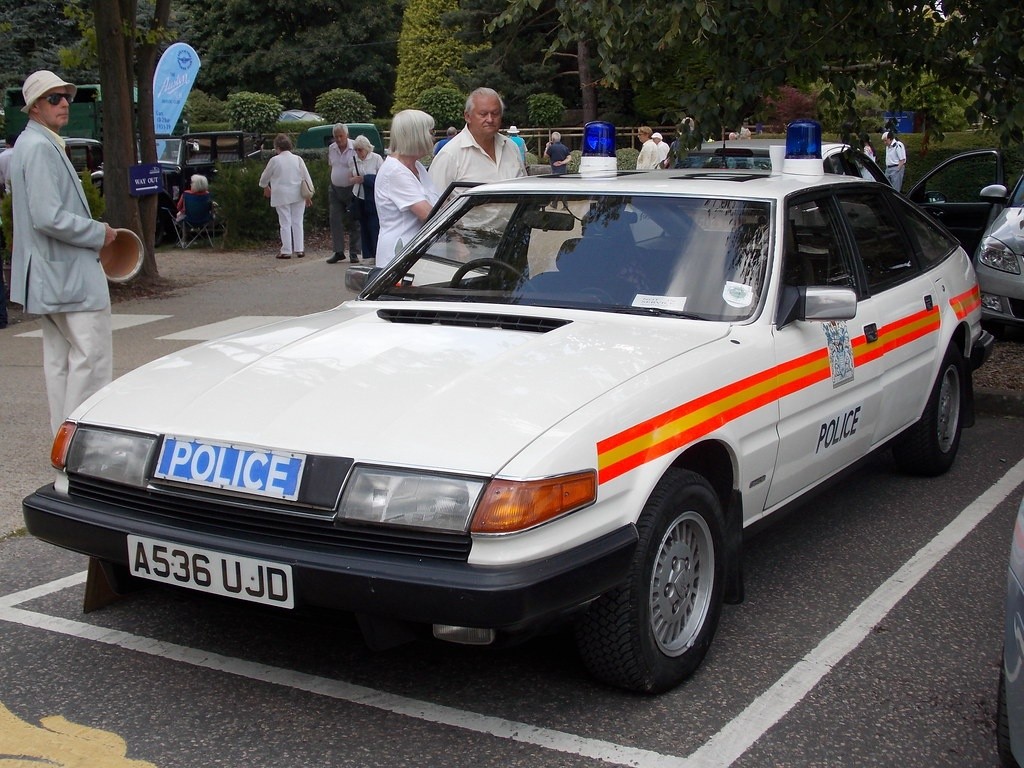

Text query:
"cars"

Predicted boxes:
[[675, 136, 1003, 251], [64, 135, 103, 194], [153, 123, 388, 247], [25, 121, 995, 693], [972, 170, 1023, 326]]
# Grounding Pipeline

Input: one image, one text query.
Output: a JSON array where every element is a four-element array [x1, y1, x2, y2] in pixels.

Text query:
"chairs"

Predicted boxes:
[[173, 191, 228, 249], [780, 220, 816, 289], [517, 236, 589, 305]]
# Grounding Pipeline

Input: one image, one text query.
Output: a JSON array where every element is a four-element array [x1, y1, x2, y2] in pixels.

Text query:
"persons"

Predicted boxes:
[[178, 174, 217, 225], [507, 126, 529, 169], [348, 135, 384, 266], [433, 126, 457, 155], [374, 110, 446, 269], [880, 132, 906, 193], [543, 132, 571, 211], [258, 135, 314, 259], [10, 70, 118, 437], [326, 123, 361, 264], [858, 133, 876, 181], [428, 88, 532, 271], [0, 136, 15, 328], [636, 117, 763, 170]]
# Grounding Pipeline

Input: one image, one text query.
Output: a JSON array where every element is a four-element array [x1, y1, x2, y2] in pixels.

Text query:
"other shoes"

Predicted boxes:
[[326, 252, 346, 264], [562, 207, 567, 211], [276, 253, 291, 259], [350, 253, 359, 263], [297, 252, 305, 258], [550, 204, 557, 209], [177, 240, 186, 247]]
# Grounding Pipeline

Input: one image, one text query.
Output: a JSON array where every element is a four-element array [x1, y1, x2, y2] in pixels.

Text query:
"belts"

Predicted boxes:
[[888, 165, 898, 168]]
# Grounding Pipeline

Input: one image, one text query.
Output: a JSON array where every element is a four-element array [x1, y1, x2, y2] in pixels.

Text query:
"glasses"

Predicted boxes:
[[431, 129, 436, 135], [40, 93, 74, 105]]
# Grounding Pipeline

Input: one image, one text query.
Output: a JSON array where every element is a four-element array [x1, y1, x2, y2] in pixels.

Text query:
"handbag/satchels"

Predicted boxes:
[[301, 177, 314, 198], [349, 197, 360, 221]]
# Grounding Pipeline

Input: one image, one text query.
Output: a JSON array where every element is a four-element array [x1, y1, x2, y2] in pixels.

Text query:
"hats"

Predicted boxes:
[[20, 70, 76, 113], [651, 132, 662, 140], [507, 126, 520, 134]]
